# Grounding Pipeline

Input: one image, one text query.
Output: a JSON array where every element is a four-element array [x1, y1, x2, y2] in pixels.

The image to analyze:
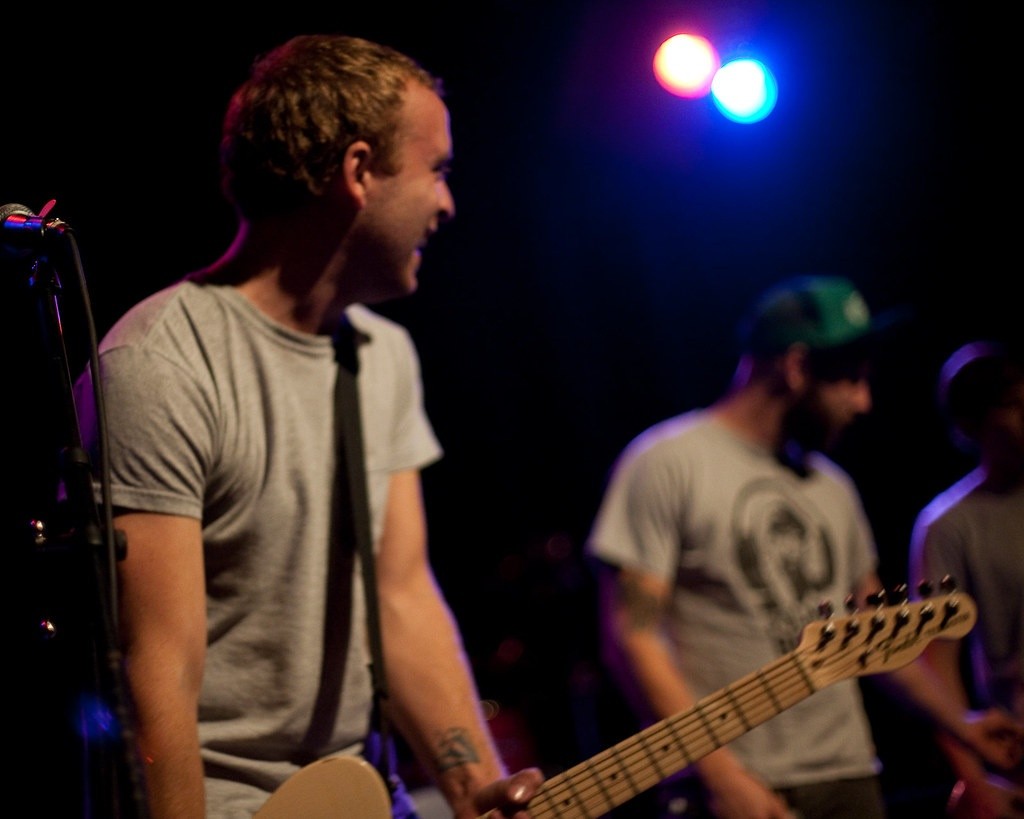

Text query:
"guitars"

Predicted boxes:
[[247, 570, 981, 818]]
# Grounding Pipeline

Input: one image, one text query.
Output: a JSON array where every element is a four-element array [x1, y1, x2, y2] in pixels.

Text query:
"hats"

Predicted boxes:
[[731, 275, 871, 348]]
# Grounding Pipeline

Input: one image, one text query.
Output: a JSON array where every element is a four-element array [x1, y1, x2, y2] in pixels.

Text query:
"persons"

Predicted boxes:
[[909, 343, 1024, 819], [78, 33, 543, 819], [585, 281, 1024, 819]]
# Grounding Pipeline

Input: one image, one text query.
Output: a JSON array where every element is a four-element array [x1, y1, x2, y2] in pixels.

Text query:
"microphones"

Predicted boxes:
[[0, 202, 74, 235]]
